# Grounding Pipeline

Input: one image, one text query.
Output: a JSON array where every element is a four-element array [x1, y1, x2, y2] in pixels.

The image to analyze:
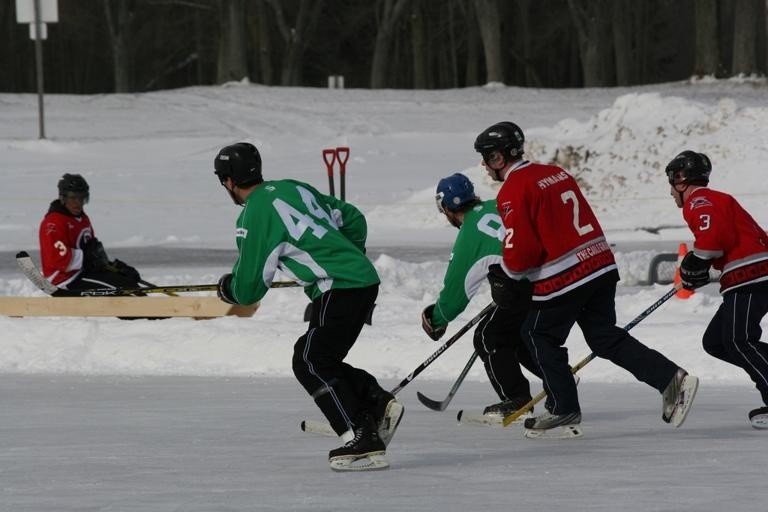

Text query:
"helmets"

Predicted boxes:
[[475, 122, 524, 162], [215, 143, 261, 185], [59, 174, 89, 207], [666, 151, 711, 183], [436, 173, 475, 214]]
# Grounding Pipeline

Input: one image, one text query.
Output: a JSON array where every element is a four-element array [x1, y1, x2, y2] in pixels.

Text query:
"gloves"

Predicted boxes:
[[487, 264, 532, 314], [422, 304, 446, 341], [112, 259, 141, 283], [218, 274, 238, 305], [680, 251, 712, 289], [81, 238, 104, 268]]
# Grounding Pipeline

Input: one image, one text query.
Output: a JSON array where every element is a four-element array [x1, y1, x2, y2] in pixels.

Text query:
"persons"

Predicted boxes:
[[215, 142, 398, 461], [40, 174, 144, 297], [475, 122, 690, 431], [421, 172, 579, 422], [665, 150, 767, 420]]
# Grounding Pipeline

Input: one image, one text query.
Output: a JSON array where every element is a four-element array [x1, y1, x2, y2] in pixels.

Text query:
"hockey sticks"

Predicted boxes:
[[15, 250, 302, 297], [300, 301, 498, 438], [417, 349, 479, 412], [457, 283, 682, 429]]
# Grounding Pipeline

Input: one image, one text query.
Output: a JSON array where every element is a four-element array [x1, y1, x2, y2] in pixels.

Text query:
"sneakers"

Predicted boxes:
[[483, 397, 534, 415], [365, 378, 397, 433], [329, 426, 386, 462], [749, 407, 768, 420], [525, 410, 581, 431], [663, 368, 688, 423]]
